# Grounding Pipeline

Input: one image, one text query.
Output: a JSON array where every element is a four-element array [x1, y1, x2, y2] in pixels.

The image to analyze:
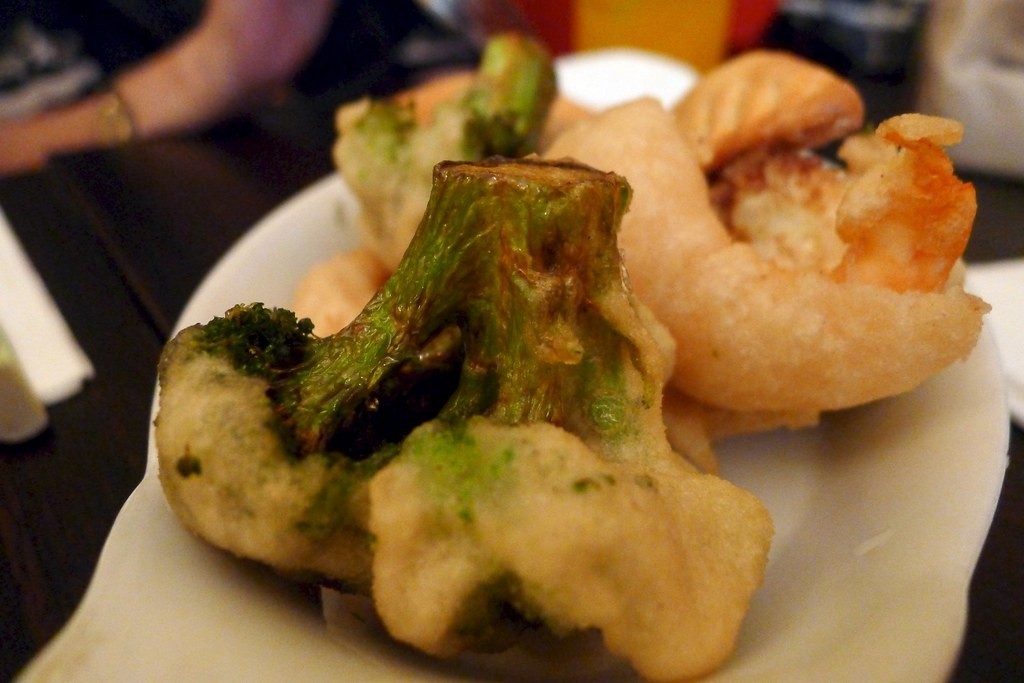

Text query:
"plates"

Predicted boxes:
[[6, 42, 1007, 683]]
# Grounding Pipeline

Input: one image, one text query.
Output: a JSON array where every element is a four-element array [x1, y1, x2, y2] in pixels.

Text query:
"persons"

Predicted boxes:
[[0, 0, 481, 175]]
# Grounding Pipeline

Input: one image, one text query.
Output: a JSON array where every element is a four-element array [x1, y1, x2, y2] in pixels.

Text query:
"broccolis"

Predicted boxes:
[[154, 160, 775, 683], [333, 38, 558, 272]]
